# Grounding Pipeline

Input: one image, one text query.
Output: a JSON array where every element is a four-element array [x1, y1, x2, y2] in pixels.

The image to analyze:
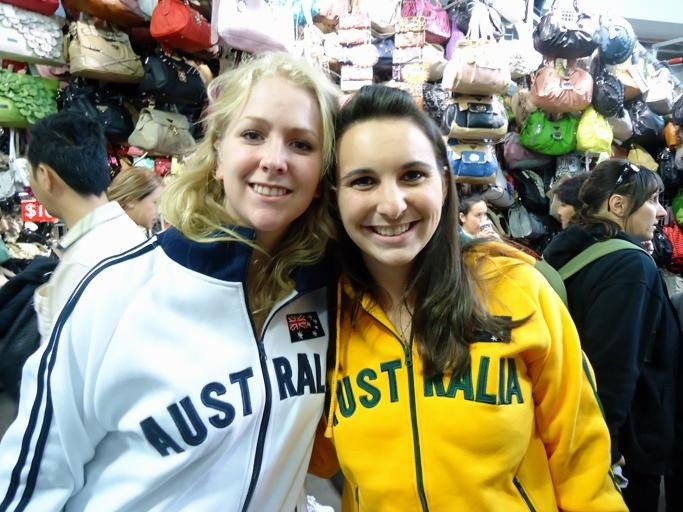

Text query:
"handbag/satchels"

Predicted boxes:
[[602, 12, 683, 275], [444, 0, 600, 242], [216, 1, 296, 56], [341, 1, 450, 119]]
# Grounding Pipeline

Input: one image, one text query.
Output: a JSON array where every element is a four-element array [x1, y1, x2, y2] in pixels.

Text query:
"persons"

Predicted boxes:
[[1, 165, 166, 439], [305, 81, 627, 512], [455, 194, 504, 249], [0, 49, 343, 512], [541, 157, 680, 512], [550, 169, 593, 232], [25, 105, 148, 347]]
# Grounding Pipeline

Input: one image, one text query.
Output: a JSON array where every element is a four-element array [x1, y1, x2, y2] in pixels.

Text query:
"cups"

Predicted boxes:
[[479, 219, 493, 232]]
